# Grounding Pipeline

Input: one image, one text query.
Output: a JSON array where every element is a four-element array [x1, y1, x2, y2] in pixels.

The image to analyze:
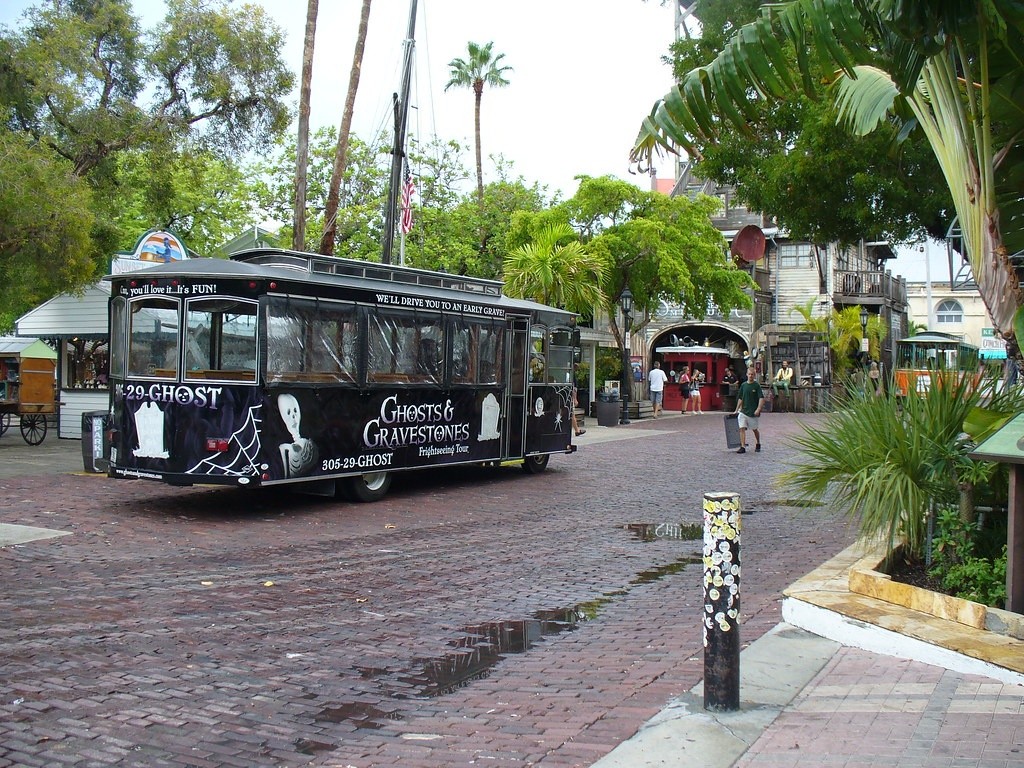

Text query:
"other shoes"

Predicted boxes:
[[774, 395, 778, 398], [691, 411, 697, 416], [737, 446, 745, 454], [696, 410, 704, 415], [786, 396, 789, 400], [755, 444, 761, 452]]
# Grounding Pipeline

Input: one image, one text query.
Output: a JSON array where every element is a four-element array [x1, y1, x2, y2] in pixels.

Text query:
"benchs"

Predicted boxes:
[[154, 368, 465, 383]]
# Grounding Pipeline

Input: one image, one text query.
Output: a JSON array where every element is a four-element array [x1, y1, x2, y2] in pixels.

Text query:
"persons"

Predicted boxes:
[[848, 361, 880, 399], [772, 360, 793, 400], [734, 368, 765, 453], [679, 366, 694, 415], [722, 368, 739, 389], [634, 368, 642, 381], [648, 361, 668, 419], [690, 369, 704, 415], [547, 376, 587, 436]]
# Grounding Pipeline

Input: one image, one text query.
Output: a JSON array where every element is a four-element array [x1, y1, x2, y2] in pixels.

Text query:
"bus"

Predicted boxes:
[[94, 247, 581, 504], [893, 331, 984, 412]]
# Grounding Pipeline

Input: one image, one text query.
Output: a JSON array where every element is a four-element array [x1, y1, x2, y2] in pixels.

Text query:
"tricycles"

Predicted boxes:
[[0, 337, 59, 446]]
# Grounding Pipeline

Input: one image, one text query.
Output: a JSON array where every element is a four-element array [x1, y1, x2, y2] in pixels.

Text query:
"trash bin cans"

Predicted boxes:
[[596, 392, 621, 427], [81, 410, 111, 472], [724, 395, 736, 412]]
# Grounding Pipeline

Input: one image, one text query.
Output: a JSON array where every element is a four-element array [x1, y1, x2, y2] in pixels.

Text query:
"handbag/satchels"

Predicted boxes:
[[689, 378, 695, 391], [678, 375, 689, 391]]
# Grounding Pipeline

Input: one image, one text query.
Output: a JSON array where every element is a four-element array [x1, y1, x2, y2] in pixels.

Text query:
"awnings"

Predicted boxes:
[[978, 350, 1007, 359]]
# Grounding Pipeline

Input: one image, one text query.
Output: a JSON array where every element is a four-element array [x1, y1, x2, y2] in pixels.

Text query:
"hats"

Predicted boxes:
[[725, 368, 729, 372]]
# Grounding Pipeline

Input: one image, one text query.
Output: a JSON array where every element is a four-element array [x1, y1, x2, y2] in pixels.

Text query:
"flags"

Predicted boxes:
[[399, 158, 417, 234]]
